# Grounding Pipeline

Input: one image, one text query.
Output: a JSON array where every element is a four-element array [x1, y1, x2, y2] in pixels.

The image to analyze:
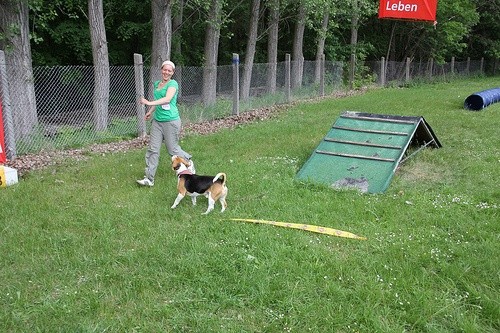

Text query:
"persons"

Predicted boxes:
[[137, 61, 197, 187]]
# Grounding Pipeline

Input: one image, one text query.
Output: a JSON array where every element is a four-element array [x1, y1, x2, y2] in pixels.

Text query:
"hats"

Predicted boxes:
[[161, 60, 175, 71]]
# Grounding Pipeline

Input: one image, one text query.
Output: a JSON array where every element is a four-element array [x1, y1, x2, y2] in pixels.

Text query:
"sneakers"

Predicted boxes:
[[136, 177, 156, 188]]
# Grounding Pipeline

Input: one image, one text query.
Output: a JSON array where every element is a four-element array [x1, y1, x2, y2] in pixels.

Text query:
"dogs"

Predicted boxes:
[[171, 155, 228, 215]]
